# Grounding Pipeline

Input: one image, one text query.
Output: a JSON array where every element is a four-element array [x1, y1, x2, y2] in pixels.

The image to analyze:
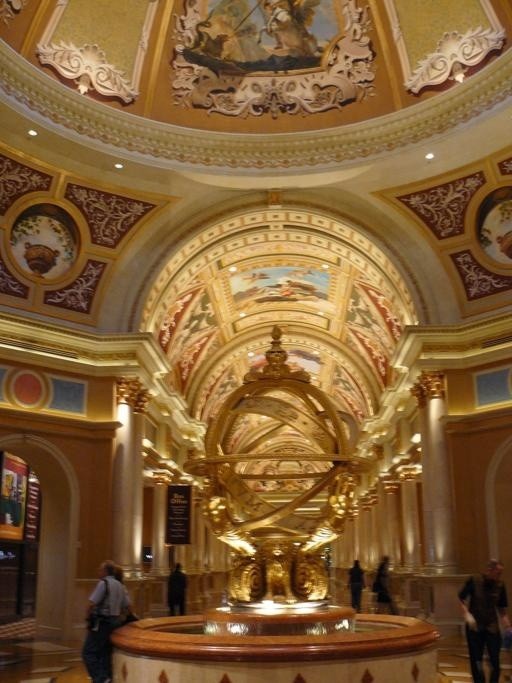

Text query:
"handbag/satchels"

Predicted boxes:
[[87, 614, 98, 630]]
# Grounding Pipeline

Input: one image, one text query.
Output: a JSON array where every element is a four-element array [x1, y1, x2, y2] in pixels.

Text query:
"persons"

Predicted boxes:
[[369, 554, 396, 615], [454, 556, 511, 683], [345, 559, 367, 613], [79, 558, 130, 680], [166, 561, 188, 616], [116, 564, 139, 630]]
